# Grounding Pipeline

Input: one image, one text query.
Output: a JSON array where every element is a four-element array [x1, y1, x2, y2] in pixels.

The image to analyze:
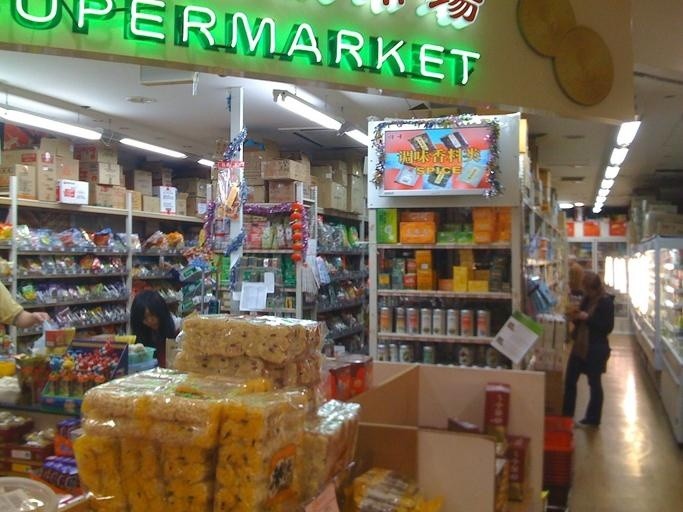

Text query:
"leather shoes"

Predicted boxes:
[[579, 417, 599, 426]]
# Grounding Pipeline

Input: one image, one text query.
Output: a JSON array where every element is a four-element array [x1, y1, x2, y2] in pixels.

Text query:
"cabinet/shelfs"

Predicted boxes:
[[369, 201, 569, 368], [244, 221, 368, 352], [343, 358, 545, 444], [130, 214, 206, 315], [1, 203, 129, 355], [1, 370, 82, 434]]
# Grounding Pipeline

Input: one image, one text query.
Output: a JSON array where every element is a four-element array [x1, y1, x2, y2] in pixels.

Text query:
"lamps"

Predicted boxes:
[[270, 88, 349, 132], [0, 87, 100, 141]]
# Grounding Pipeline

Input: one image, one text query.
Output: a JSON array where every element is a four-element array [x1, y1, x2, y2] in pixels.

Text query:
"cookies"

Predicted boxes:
[[517, 0, 576, 59], [554, 27, 614, 105]]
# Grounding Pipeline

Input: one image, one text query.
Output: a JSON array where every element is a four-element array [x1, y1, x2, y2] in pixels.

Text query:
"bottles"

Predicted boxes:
[[374, 306, 503, 367]]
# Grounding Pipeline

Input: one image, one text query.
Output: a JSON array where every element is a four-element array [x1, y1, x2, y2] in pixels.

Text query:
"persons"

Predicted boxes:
[[130, 290, 183, 369], [565, 255, 584, 343], [0, 281, 50, 328], [561, 272, 616, 428]]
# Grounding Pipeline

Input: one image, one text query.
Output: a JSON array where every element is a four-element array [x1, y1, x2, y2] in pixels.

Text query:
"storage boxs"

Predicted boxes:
[[2, 129, 210, 217], [546, 415, 575, 445], [244, 140, 365, 215], [567, 179, 682, 243], [393, 104, 567, 231]]
